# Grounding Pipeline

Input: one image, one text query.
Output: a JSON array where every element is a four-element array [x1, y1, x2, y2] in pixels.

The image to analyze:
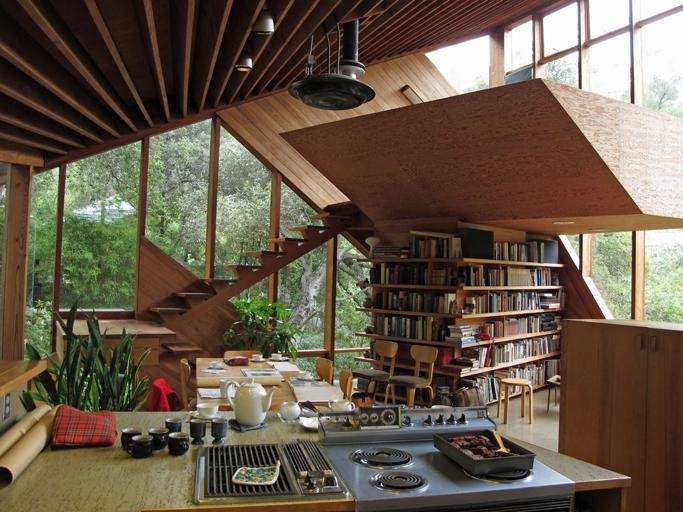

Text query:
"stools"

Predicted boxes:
[[497, 378, 533, 423], [547, 374, 561, 412]]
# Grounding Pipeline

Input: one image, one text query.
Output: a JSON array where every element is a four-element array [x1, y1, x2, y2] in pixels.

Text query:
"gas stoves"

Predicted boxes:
[[316, 438, 576, 512]]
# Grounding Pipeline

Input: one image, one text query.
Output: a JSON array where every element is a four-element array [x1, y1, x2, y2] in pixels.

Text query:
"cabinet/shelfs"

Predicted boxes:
[[352, 233, 563, 420]]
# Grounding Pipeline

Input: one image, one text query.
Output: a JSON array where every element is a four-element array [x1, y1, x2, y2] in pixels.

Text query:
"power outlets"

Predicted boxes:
[[4, 393, 12, 419]]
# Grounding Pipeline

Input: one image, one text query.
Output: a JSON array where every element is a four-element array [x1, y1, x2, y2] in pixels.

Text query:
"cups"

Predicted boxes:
[[281, 401, 300, 420], [120, 427, 143, 451], [125, 434, 154, 458], [167, 431, 190, 456], [252, 354, 263, 360], [298, 370, 312, 378], [196, 402, 220, 417], [328, 399, 355, 411], [148, 428, 170, 450], [164, 418, 182, 432], [210, 360, 222, 368], [271, 353, 282, 359]]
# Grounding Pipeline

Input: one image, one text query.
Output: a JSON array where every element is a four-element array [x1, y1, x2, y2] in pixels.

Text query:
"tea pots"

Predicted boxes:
[[225, 377, 277, 425]]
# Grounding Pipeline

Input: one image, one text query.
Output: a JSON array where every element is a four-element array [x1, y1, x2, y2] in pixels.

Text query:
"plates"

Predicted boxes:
[[297, 416, 330, 430], [250, 360, 265, 363], [189, 411, 225, 420], [269, 359, 290, 362]]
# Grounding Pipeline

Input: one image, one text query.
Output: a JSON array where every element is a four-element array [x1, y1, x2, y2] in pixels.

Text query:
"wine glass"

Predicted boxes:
[[189, 419, 207, 445], [211, 417, 228, 444]]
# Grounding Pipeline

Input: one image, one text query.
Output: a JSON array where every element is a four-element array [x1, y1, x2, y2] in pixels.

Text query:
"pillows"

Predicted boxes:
[[53, 403, 116, 449]]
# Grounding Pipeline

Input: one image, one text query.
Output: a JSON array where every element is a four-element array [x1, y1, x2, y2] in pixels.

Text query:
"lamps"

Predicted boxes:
[[234, 55, 255, 72], [252, 10, 274, 37], [288, 20, 375, 110]]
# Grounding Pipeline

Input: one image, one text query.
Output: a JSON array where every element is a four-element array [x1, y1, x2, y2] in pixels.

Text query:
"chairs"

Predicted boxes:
[[354, 340, 399, 407], [389, 344, 438, 408], [180, 359, 197, 410], [312, 371, 352, 411], [224, 350, 262, 361], [315, 357, 334, 384]]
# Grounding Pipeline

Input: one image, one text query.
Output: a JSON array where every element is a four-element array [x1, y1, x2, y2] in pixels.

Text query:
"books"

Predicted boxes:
[[357, 230, 566, 406], [240, 368, 285, 382], [288, 377, 343, 403]]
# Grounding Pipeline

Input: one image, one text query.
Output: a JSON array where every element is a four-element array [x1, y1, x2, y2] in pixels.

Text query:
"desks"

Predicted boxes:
[[196, 357, 349, 411]]
